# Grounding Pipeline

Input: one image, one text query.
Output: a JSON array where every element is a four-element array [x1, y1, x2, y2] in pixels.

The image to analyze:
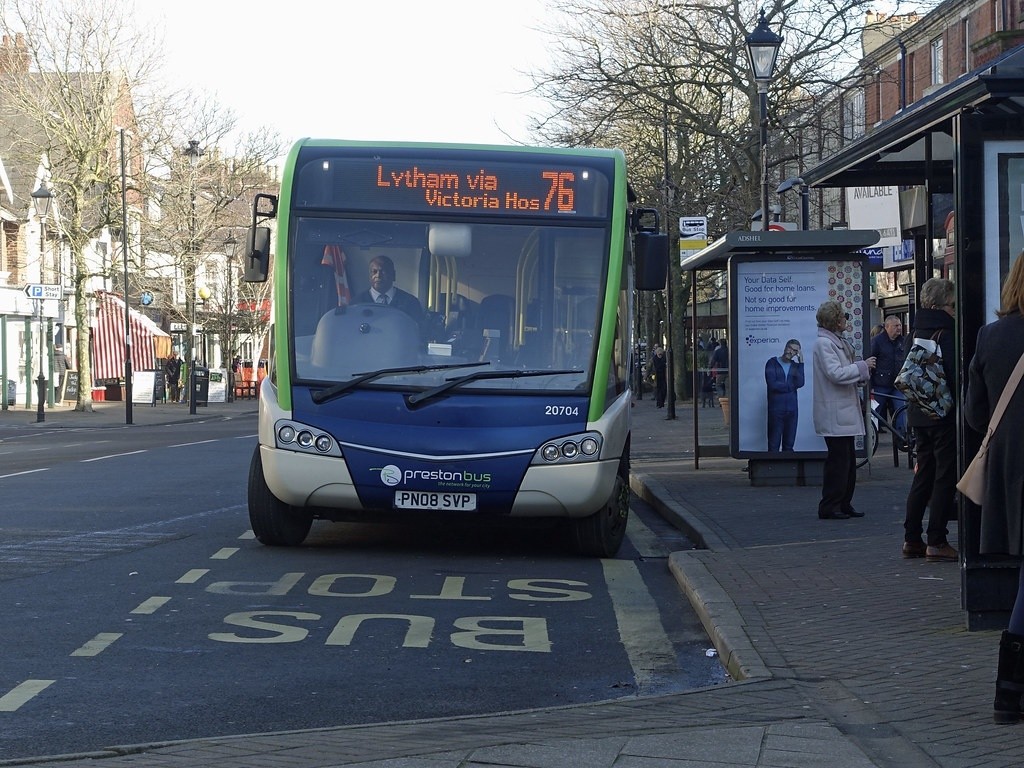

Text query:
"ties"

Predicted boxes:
[[381, 295, 387, 304]]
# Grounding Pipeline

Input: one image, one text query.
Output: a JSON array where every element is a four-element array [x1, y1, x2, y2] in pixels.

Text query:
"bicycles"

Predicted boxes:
[[856, 391, 915, 468]]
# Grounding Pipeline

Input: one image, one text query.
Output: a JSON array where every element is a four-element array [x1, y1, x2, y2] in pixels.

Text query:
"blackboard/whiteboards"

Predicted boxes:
[[62, 370, 79, 402], [132, 371, 156, 404], [145, 369, 166, 400]]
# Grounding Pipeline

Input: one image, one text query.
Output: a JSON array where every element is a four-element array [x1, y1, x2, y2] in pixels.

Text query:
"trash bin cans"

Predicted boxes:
[[0, 379, 17, 405], [189, 367, 209, 407]]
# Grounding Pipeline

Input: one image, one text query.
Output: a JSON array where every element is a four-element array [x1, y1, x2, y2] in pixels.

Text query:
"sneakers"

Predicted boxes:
[[902, 540, 927, 559], [925, 541, 958, 561]]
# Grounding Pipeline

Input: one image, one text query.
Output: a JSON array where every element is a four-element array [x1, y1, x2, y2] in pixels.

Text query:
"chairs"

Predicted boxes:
[[234, 373, 257, 400], [438, 294, 598, 364]]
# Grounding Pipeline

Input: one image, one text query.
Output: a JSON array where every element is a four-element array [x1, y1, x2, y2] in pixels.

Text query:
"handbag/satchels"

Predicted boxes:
[[955, 446, 989, 505], [894, 329, 955, 421]]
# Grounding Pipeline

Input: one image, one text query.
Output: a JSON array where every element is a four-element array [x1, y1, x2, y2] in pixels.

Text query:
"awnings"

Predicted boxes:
[[99, 293, 173, 358]]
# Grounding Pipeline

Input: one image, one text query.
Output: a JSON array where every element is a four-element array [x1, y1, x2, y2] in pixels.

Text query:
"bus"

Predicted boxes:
[[244, 137, 669, 560]]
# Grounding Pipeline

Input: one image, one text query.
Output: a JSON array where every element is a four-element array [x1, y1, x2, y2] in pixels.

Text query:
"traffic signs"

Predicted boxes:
[[23, 283, 63, 300]]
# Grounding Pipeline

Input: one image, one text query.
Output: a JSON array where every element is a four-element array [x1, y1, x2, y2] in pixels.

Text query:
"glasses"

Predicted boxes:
[[947, 302, 955, 308], [843, 313, 849, 320]]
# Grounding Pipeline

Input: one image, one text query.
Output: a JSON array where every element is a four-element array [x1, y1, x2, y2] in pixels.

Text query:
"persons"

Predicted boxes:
[[872, 313, 911, 438], [812, 302, 877, 518], [53, 344, 70, 405], [963, 249, 1024, 724], [707, 337, 718, 349], [697, 336, 703, 348], [646, 346, 667, 408], [766, 338, 804, 451], [903, 278, 961, 562], [166, 351, 188, 404], [348, 255, 425, 329], [702, 362, 718, 410], [232, 355, 242, 373]]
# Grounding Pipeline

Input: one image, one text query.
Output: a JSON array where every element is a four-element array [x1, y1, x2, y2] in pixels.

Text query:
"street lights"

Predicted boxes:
[[29, 178, 54, 423], [223, 229, 239, 402], [181, 140, 208, 414], [198, 287, 211, 368], [742, 6, 786, 231]]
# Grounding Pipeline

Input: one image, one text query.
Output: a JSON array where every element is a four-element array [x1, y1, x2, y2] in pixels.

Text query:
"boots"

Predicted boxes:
[[991, 630, 1024, 724]]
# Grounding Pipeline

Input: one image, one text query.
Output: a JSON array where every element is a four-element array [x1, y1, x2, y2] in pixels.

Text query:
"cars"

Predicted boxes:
[[235, 359, 269, 398]]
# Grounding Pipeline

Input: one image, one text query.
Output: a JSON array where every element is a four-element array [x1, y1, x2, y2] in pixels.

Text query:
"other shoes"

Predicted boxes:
[[897, 436, 907, 452], [819, 506, 850, 519], [656, 402, 662, 408], [843, 503, 865, 518]]
